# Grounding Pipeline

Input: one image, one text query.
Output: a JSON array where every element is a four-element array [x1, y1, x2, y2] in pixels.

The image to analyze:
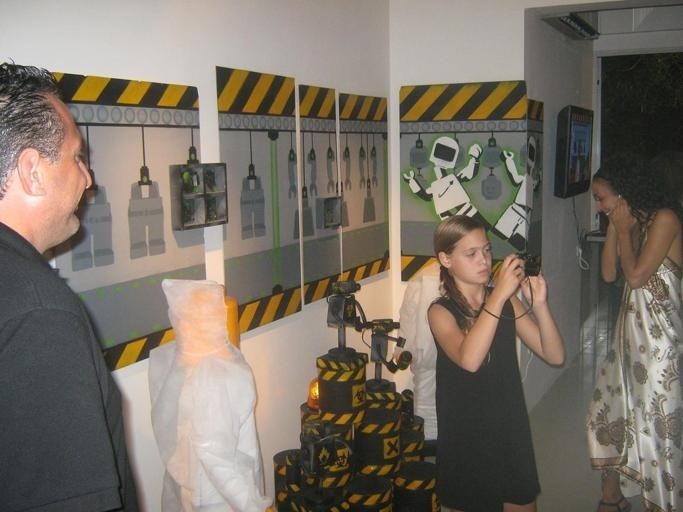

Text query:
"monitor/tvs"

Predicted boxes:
[[555, 105, 593, 198]]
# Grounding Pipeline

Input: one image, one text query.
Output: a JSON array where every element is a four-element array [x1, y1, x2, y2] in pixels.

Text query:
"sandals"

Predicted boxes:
[[597, 495, 632, 512]]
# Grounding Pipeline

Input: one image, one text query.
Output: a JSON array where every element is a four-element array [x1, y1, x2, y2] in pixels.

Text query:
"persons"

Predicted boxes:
[[424, 213, 568, 511], [0, 62, 136, 512], [582, 152, 682, 511]]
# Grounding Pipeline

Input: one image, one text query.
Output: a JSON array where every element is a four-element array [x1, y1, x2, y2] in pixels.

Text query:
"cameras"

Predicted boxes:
[[525, 257, 540, 275]]
